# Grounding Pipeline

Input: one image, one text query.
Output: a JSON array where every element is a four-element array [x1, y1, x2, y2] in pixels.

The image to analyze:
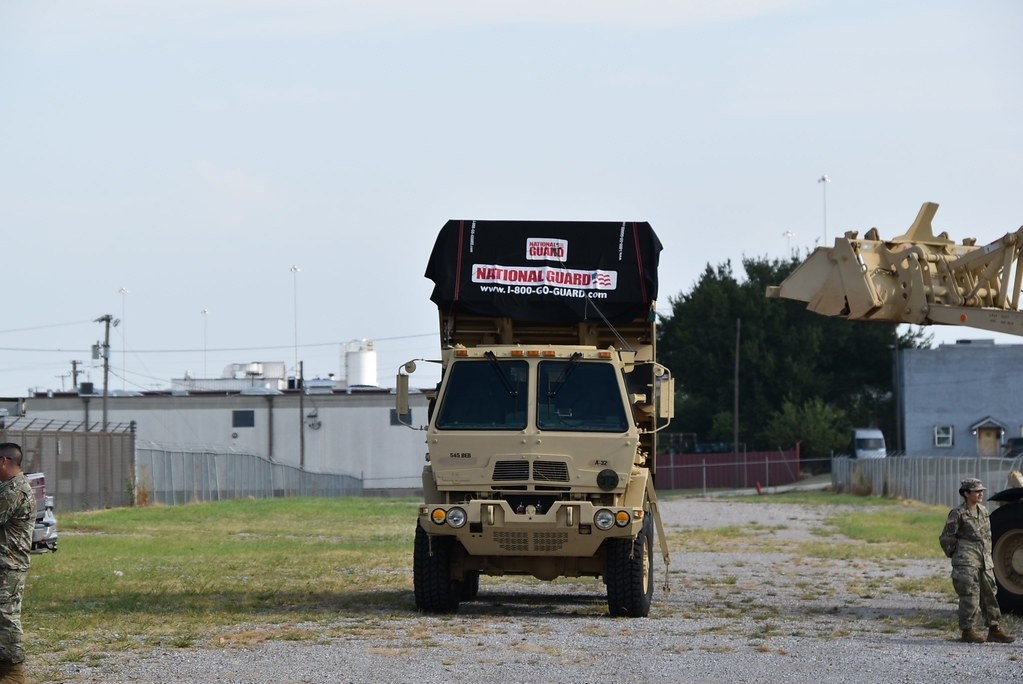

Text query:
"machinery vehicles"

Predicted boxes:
[[766, 201, 1023, 614]]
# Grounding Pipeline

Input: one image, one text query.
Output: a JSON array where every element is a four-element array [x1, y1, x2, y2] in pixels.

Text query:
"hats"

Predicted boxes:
[[960, 478, 987, 492]]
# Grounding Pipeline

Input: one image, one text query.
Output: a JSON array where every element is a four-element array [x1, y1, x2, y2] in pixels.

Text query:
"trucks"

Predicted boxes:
[[395, 219, 675, 618]]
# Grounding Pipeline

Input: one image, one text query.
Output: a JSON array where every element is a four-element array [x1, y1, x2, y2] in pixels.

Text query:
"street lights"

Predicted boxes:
[[121, 288, 130, 392], [290, 265, 301, 376], [783, 229, 796, 264], [200, 309, 209, 378], [817, 174, 830, 249]]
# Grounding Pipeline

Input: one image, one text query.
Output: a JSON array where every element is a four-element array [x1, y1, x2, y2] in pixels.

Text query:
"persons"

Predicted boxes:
[[938, 478, 1016, 642], [0, 443, 38, 684]]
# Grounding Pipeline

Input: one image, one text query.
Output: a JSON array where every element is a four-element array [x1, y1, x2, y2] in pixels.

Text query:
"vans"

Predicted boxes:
[[850, 427, 887, 460]]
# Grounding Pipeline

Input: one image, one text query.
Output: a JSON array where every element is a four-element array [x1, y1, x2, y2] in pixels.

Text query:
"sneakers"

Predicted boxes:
[[962, 629, 984, 643], [987, 627, 1015, 642]]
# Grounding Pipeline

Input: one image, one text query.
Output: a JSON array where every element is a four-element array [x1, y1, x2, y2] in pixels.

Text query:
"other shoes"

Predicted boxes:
[[0, 662, 24, 684]]
